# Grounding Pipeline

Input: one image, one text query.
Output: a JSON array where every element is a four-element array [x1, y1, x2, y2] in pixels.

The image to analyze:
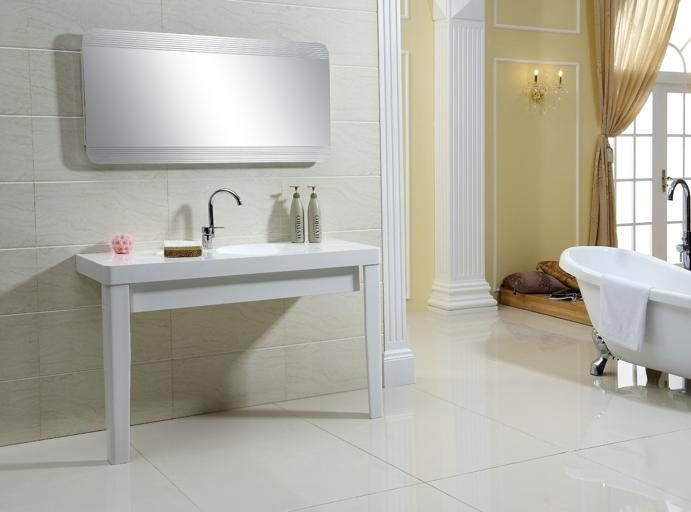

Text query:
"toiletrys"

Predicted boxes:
[[308, 185, 321, 243], [290, 186, 304, 243]]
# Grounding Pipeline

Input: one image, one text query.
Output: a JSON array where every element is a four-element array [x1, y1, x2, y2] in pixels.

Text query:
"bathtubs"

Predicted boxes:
[[558, 246, 690, 379]]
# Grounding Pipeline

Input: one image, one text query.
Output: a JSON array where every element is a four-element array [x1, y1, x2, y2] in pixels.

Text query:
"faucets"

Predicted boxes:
[[668, 179, 691, 232], [209, 188, 244, 226]]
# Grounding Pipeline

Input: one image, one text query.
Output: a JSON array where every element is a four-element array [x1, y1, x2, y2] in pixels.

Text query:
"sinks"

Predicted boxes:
[[214, 244, 298, 256]]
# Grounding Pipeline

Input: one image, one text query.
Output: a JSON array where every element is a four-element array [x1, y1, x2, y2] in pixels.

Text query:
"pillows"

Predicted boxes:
[[500, 271, 578, 298], [535, 261, 581, 294]]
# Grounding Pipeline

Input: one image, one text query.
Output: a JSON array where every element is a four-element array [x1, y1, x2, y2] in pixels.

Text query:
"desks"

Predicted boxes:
[[75, 239, 383, 465]]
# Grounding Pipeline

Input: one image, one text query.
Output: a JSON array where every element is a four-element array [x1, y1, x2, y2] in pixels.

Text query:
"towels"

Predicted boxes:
[[598, 272, 654, 353]]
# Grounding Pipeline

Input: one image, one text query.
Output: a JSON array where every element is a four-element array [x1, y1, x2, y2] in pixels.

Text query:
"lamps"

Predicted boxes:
[[523, 65, 567, 116]]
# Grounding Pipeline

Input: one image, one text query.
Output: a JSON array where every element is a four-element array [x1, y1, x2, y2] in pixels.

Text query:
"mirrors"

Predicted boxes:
[[81, 28, 331, 166]]
